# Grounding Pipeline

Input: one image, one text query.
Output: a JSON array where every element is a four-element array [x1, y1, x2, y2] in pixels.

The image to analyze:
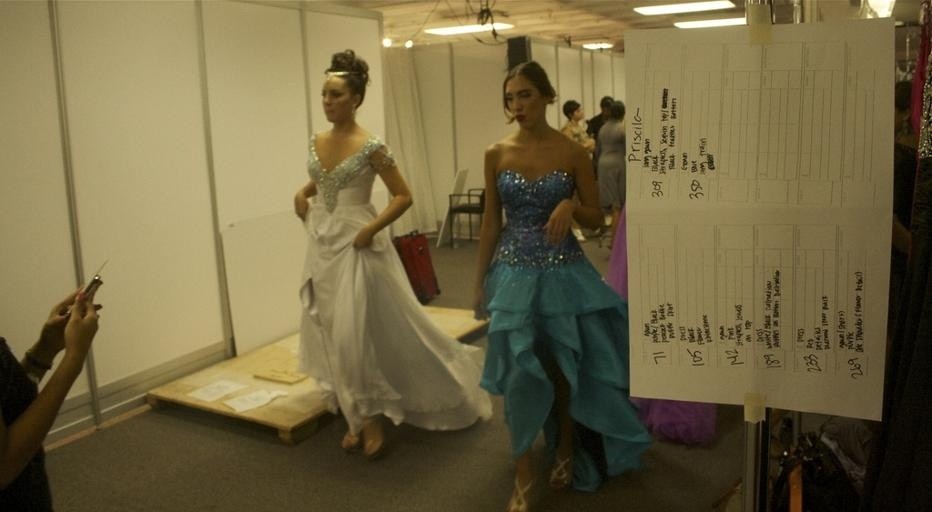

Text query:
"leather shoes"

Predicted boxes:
[[342, 434, 382, 457]]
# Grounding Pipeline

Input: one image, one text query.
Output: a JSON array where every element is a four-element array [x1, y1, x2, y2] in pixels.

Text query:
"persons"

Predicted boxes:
[[472, 58, 655, 511], [293, 48, 495, 460], [892, 80, 914, 275], [0, 283, 104, 512], [587, 96, 614, 181], [560, 100, 596, 160], [595, 100, 627, 213]]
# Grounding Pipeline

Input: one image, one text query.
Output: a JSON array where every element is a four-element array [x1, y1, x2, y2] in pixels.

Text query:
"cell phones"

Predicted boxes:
[[64, 274, 101, 314]]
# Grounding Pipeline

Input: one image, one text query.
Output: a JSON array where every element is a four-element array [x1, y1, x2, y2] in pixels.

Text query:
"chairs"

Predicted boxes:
[[448, 188, 485, 249]]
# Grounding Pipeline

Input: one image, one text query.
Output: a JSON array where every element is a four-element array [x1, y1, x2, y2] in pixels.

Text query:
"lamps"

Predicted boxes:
[[858, 0, 896, 19], [633, 0, 736, 16], [672, 11, 746, 29], [424, 15, 514, 35], [575, 39, 615, 49]]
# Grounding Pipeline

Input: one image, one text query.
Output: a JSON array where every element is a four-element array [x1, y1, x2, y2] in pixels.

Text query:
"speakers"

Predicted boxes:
[[508, 35, 532, 69]]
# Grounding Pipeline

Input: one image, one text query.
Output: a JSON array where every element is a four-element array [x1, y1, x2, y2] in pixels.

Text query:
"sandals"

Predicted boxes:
[[507, 475, 532, 512], [549, 456, 572, 489]]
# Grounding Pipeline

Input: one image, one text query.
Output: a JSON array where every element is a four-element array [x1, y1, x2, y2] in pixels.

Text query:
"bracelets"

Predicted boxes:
[[26, 349, 52, 370]]
